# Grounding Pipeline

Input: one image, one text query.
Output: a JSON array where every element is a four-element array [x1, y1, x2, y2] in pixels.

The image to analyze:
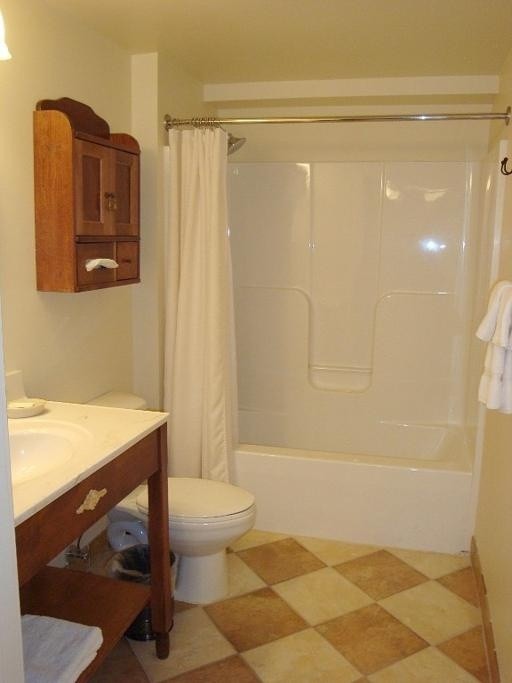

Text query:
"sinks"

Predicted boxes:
[[9, 418, 93, 492]]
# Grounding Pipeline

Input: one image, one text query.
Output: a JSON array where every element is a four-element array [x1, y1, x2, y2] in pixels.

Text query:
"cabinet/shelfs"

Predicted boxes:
[[32, 97, 140, 294], [14, 423, 172, 683]]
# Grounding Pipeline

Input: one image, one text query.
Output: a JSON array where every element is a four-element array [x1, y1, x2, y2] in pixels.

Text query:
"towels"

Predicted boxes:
[[475, 280, 512, 414], [21, 614, 104, 683]]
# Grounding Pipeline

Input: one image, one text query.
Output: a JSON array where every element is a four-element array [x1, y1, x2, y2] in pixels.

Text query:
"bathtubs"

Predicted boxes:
[[231, 411, 475, 555]]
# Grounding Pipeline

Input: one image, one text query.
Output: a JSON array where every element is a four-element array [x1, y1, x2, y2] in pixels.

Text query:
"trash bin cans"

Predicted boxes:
[[111, 544, 179, 641]]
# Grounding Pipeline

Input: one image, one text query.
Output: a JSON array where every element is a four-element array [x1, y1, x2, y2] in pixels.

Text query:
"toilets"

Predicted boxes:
[[81, 389, 255, 606]]
